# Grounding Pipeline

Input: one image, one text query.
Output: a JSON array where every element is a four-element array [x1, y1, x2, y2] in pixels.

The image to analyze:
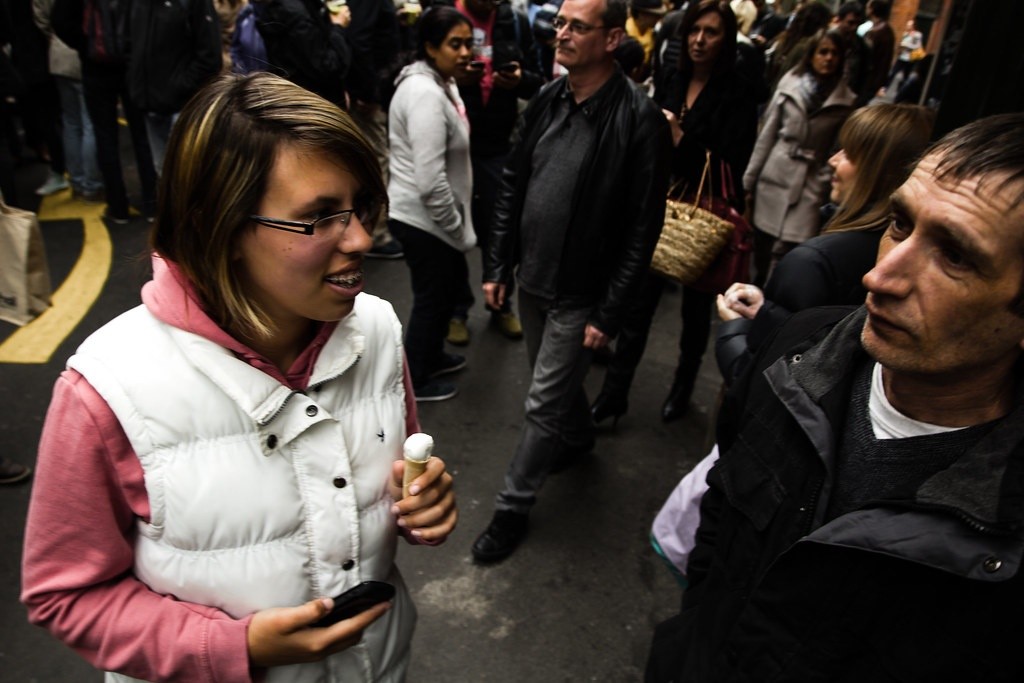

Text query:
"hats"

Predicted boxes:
[[627, 0, 667, 14]]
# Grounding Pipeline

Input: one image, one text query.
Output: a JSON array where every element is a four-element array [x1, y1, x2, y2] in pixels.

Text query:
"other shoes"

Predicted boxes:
[[103, 206, 130, 225], [447, 314, 471, 343], [494, 310, 523, 339], [35, 175, 69, 196], [85, 194, 107, 201]]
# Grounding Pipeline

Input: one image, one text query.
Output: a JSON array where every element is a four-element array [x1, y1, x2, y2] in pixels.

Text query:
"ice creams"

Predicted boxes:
[[402, 433, 434, 499]]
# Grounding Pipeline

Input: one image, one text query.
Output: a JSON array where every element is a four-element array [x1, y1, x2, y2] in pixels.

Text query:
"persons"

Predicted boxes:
[[715, 104, 944, 381], [469, 0, 674, 566], [0, 0, 924, 429], [17, 70, 458, 683], [386, 7, 478, 401], [643, 112, 1024, 683]]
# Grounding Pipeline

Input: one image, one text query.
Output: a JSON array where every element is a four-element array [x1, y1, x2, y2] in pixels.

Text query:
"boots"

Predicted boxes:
[[660, 292, 711, 424], [589, 320, 653, 427]]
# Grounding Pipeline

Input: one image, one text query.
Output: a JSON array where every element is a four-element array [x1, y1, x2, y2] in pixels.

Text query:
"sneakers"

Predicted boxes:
[[411, 354, 466, 402], [363, 239, 405, 259]]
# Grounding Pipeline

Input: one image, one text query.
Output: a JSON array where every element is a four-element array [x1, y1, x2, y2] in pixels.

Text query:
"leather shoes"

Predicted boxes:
[[0, 455, 32, 486], [551, 438, 595, 472], [472, 508, 530, 561]]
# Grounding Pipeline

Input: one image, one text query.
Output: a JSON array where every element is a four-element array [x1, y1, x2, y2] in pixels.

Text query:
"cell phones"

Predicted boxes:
[[307, 581, 397, 628], [471, 61, 485, 74], [498, 63, 518, 75]]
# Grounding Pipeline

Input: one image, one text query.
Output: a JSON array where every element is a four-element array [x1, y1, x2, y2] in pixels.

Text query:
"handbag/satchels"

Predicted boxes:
[[648, 147, 756, 298], [0, 191, 52, 327], [911, 48, 926, 62], [82, 0, 132, 66]]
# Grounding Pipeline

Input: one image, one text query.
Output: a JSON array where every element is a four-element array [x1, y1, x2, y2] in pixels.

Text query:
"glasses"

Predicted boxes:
[[249, 201, 390, 243], [552, 18, 605, 34]]
